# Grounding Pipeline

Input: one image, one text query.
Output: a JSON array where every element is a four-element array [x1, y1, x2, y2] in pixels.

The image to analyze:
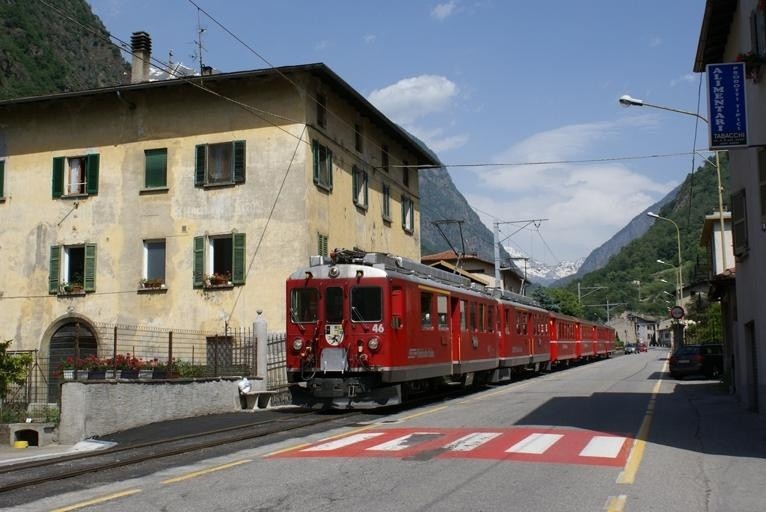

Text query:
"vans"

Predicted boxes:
[[670, 343, 724, 380]]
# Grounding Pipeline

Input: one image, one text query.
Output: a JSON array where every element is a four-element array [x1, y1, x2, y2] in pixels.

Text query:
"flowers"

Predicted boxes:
[[51, 351, 177, 382], [734, 50, 765, 78], [199, 269, 234, 297]]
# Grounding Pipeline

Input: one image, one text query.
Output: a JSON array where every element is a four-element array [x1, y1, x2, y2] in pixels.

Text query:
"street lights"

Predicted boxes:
[[646, 211, 684, 311], [617, 93, 728, 275], [655, 259, 678, 312]]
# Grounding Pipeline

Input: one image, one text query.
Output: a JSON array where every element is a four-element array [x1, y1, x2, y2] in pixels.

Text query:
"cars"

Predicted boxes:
[[625, 343, 640, 353], [638, 343, 648, 352]]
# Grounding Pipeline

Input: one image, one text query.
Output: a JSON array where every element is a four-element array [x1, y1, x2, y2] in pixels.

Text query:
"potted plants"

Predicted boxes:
[[141, 278, 162, 291], [56, 280, 83, 295]]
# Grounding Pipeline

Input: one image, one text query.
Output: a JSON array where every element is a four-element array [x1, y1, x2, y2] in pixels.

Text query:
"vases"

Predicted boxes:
[[211, 279, 227, 286]]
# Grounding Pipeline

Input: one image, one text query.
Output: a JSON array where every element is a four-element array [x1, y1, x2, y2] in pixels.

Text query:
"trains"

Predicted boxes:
[[285, 247, 618, 414]]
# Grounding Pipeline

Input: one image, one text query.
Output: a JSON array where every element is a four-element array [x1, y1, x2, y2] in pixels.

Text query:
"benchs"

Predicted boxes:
[[242, 389, 279, 410]]
